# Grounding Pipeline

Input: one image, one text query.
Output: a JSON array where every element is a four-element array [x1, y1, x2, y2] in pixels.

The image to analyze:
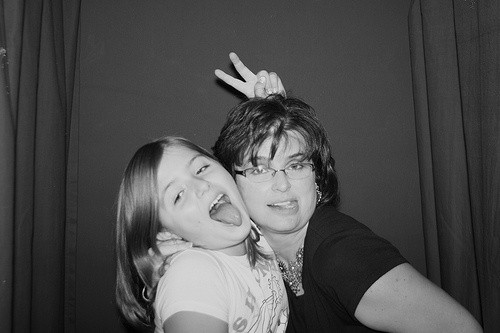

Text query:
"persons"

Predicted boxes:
[[111, 49, 290, 331], [213, 91, 485, 333]]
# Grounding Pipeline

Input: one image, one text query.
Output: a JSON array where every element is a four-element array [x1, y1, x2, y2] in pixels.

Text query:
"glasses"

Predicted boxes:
[[234, 163, 317, 183]]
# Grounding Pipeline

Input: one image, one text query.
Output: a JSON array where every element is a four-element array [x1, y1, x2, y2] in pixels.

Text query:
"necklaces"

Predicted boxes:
[[272, 248, 305, 293]]
